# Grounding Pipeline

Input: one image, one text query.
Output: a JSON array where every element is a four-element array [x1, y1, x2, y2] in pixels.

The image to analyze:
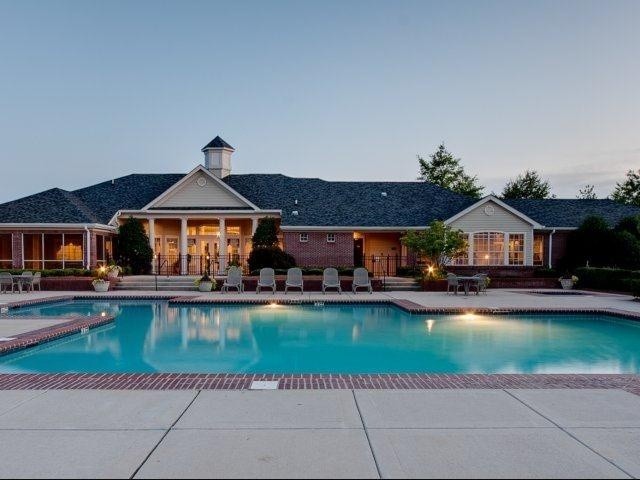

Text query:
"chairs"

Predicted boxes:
[[220, 266, 375, 295], [446, 271, 489, 297], [0, 271, 41, 294]]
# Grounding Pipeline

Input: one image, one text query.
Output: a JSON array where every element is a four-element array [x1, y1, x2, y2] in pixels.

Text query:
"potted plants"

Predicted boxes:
[[559, 271, 578, 289], [91, 278, 111, 292]]
